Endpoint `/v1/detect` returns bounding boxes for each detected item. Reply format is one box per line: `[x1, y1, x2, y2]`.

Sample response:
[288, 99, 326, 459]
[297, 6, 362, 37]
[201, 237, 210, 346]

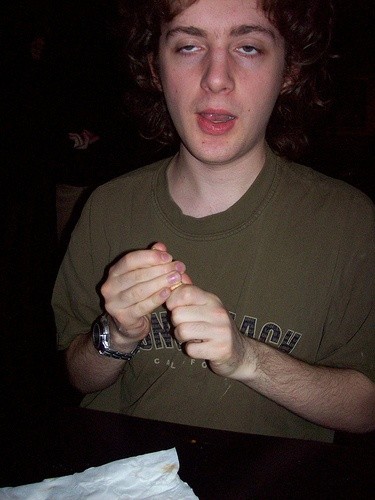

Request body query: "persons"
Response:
[51, 1, 375, 445]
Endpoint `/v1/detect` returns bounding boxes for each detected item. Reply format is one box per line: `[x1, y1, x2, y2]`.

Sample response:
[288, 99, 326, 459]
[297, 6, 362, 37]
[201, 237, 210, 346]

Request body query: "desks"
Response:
[0, 400, 375, 500]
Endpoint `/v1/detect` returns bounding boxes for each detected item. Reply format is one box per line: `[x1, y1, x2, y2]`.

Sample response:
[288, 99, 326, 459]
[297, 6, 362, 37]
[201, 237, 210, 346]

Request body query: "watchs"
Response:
[90, 313, 144, 361]
